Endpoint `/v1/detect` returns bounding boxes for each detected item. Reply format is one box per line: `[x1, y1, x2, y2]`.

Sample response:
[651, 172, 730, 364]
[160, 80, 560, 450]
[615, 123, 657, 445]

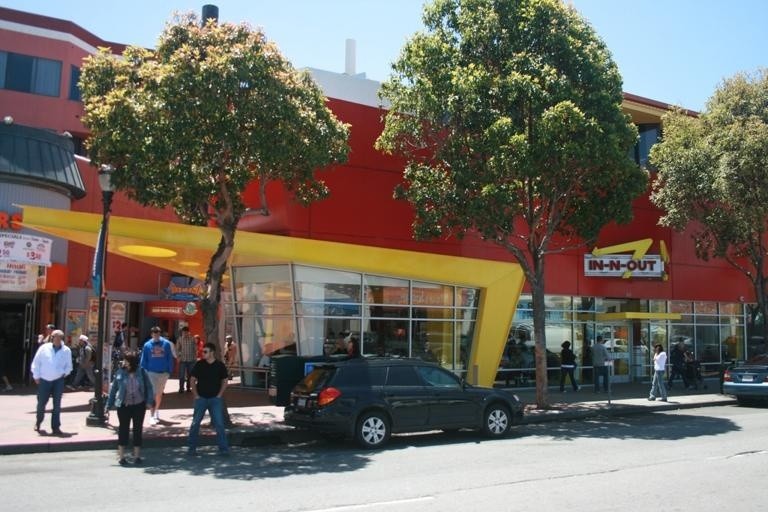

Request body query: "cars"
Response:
[281, 356, 524, 450]
[720, 352, 767, 407]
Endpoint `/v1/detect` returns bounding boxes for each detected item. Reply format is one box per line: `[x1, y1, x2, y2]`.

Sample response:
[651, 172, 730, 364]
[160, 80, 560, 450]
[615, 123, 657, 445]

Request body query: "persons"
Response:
[65, 335, 97, 392]
[224, 335, 237, 380]
[194, 334, 204, 362]
[0, 346, 13, 392]
[504, 334, 529, 385]
[667, 337, 692, 389]
[30, 329, 73, 435]
[162, 332, 170, 341]
[105, 349, 153, 465]
[344, 340, 362, 361]
[38, 324, 55, 345]
[188, 343, 232, 456]
[590, 335, 611, 394]
[560, 341, 581, 393]
[647, 344, 668, 401]
[110, 322, 128, 383]
[175, 327, 198, 394]
[140, 326, 174, 425]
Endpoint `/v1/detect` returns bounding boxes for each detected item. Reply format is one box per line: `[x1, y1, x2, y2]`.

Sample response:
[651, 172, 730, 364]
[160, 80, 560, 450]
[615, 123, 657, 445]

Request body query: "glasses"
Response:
[202, 349, 212, 354]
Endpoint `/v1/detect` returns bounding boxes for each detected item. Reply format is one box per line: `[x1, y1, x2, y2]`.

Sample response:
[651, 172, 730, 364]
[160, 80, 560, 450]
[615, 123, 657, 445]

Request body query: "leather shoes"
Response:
[53, 429, 67, 436]
[34, 424, 40, 430]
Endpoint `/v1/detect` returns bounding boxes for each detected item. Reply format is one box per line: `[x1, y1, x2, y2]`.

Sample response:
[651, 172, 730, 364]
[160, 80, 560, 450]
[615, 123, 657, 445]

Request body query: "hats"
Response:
[80, 334, 88, 342]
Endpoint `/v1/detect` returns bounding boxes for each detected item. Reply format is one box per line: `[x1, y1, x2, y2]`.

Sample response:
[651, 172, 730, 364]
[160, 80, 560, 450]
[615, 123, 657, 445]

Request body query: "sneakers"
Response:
[150, 417, 156, 426]
[218, 448, 232, 456]
[187, 447, 197, 454]
[134, 457, 143, 463]
[154, 417, 162, 424]
[118, 458, 129, 466]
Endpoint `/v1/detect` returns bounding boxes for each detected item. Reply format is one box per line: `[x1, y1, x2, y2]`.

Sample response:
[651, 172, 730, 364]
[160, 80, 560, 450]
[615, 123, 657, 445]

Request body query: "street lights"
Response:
[86, 162, 119, 425]
